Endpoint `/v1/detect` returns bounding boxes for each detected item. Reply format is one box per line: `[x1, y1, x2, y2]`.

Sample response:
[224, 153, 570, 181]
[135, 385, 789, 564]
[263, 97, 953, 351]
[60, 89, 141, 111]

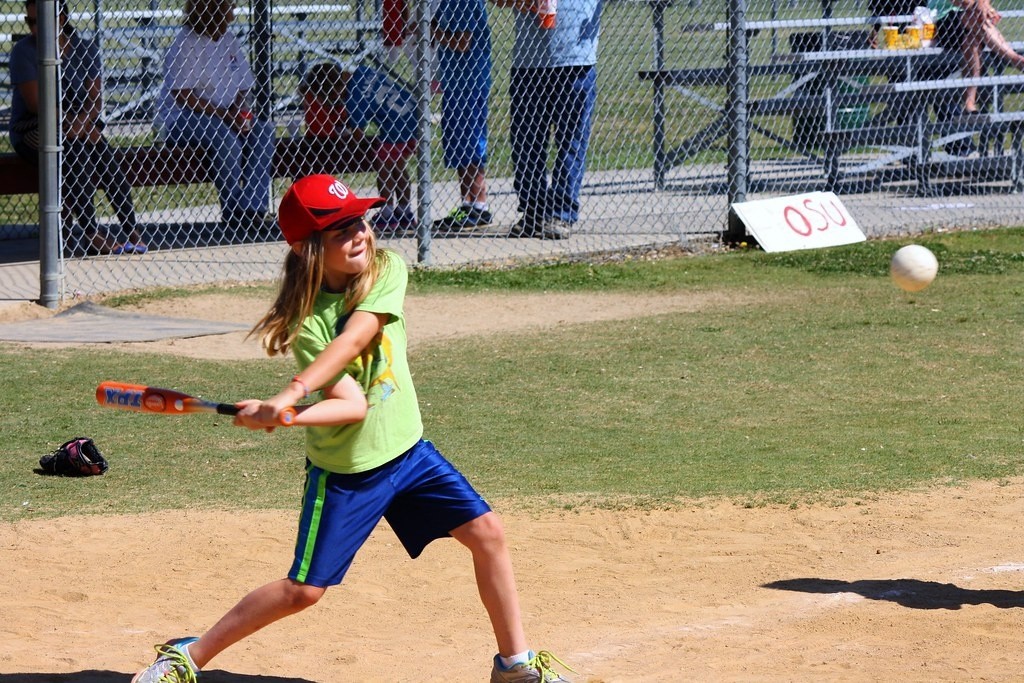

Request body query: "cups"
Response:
[882, 27, 898, 50]
[906, 25, 921, 49]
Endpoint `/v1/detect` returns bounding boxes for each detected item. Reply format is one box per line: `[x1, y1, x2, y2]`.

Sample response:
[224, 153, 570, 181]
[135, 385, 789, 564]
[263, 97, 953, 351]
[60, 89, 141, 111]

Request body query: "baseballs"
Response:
[891, 245, 939, 293]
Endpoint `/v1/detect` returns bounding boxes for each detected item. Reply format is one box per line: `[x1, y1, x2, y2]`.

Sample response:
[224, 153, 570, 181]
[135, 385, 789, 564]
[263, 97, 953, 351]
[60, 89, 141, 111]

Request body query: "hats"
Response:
[279, 173, 387, 245]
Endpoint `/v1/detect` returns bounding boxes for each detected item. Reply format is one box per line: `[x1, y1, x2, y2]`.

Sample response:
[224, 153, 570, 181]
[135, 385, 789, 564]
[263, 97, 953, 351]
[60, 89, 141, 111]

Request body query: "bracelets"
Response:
[291, 376, 310, 399]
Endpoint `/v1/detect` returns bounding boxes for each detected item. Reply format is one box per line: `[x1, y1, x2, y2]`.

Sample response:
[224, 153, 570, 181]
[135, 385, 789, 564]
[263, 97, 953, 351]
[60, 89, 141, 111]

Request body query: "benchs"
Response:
[680, 9, 1024, 191]
[0, 135, 414, 237]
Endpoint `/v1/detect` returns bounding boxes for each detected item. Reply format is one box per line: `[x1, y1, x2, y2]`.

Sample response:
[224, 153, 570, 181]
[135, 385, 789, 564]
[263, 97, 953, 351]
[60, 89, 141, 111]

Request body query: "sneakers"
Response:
[490, 650, 579, 683]
[431, 203, 493, 230]
[368, 210, 418, 234]
[131, 637, 202, 683]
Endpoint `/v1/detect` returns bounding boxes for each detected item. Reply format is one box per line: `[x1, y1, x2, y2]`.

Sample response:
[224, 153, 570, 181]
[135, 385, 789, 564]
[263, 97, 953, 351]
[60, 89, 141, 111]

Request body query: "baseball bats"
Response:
[97, 382, 297, 428]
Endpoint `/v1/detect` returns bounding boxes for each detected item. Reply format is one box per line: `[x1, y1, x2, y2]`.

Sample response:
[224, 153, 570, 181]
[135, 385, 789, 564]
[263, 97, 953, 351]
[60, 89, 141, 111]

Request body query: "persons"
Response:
[406, 0, 604, 239]
[9, 0, 148, 253]
[307, 63, 418, 229]
[154, 0, 276, 230]
[129, 173, 603, 683]
[928, 0, 1024, 113]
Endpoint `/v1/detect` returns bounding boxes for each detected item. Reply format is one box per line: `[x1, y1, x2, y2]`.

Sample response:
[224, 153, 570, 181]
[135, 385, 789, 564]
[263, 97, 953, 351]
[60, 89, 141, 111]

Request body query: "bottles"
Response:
[240, 91, 254, 132]
[537, 0, 557, 29]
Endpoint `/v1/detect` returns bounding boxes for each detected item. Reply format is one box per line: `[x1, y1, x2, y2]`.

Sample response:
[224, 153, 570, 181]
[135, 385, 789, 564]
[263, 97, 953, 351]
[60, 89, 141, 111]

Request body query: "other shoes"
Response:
[542, 218, 572, 239]
[508, 216, 544, 238]
[220, 207, 286, 244]
[85, 242, 134, 255]
[133, 245, 147, 252]
